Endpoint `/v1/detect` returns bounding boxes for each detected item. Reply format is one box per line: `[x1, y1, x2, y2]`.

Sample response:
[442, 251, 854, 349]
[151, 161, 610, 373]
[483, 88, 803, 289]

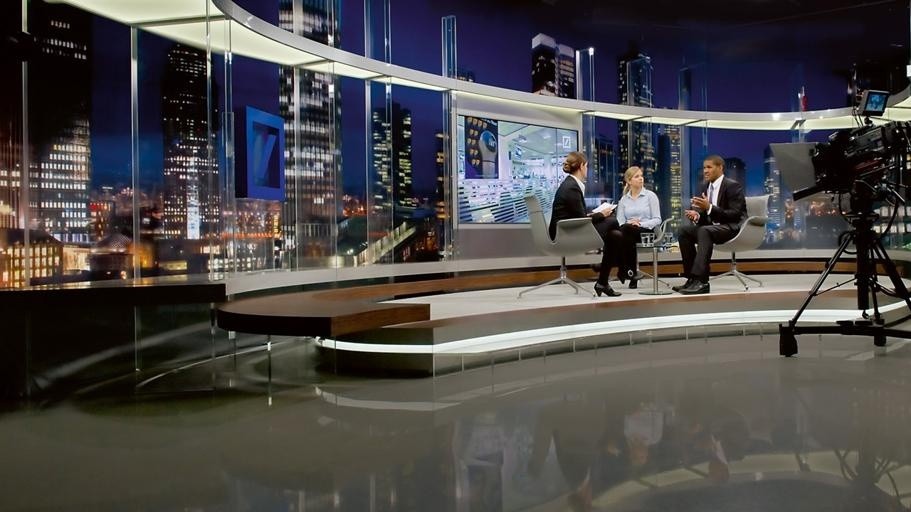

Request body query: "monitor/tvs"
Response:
[452, 113, 582, 231]
[859, 90, 889, 117]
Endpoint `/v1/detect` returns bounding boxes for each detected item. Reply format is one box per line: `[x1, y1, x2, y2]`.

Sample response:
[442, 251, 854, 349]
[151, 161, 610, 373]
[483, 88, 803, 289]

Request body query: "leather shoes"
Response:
[679, 280, 712, 295]
[618, 272, 638, 289]
[672, 277, 694, 292]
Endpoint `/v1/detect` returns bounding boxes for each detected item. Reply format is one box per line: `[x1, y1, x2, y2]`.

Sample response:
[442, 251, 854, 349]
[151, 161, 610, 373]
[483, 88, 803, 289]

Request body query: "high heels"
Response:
[594, 281, 623, 297]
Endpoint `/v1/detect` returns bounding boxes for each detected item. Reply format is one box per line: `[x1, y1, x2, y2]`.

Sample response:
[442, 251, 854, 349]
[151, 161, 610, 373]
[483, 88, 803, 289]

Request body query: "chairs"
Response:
[608, 216, 675, 288]
[518, 195, 605, 298]
[708, 193, 775, 288]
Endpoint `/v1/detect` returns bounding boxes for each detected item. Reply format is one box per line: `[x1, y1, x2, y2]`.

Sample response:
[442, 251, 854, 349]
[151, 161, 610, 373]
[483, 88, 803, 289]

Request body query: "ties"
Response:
[708, 185, 714, 223]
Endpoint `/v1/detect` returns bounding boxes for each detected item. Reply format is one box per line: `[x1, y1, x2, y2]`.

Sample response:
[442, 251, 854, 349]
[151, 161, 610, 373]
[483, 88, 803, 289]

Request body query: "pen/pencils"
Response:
[610, 201, 614, 208]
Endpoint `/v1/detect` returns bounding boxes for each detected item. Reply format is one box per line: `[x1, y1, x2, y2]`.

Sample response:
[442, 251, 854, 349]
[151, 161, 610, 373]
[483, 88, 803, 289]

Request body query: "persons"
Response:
[549, 152, 645, 297]
[615, 166, 662, 289]
[866, 95, 883, 111]
[672, 155, 748, 294]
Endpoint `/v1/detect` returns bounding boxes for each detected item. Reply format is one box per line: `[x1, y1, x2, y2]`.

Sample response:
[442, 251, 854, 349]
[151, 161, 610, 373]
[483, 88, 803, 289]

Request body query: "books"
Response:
[592, 202, 617, 214]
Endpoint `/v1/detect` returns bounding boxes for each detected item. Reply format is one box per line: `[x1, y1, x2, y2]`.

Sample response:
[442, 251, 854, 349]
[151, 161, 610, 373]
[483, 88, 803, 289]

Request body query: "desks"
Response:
[636, 246, 678, 295]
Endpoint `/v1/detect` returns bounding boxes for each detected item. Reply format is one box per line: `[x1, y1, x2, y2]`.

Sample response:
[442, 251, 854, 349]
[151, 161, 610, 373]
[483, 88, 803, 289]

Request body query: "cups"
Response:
[664, 233, 674, 245]
[641, 233, 655, 247]
